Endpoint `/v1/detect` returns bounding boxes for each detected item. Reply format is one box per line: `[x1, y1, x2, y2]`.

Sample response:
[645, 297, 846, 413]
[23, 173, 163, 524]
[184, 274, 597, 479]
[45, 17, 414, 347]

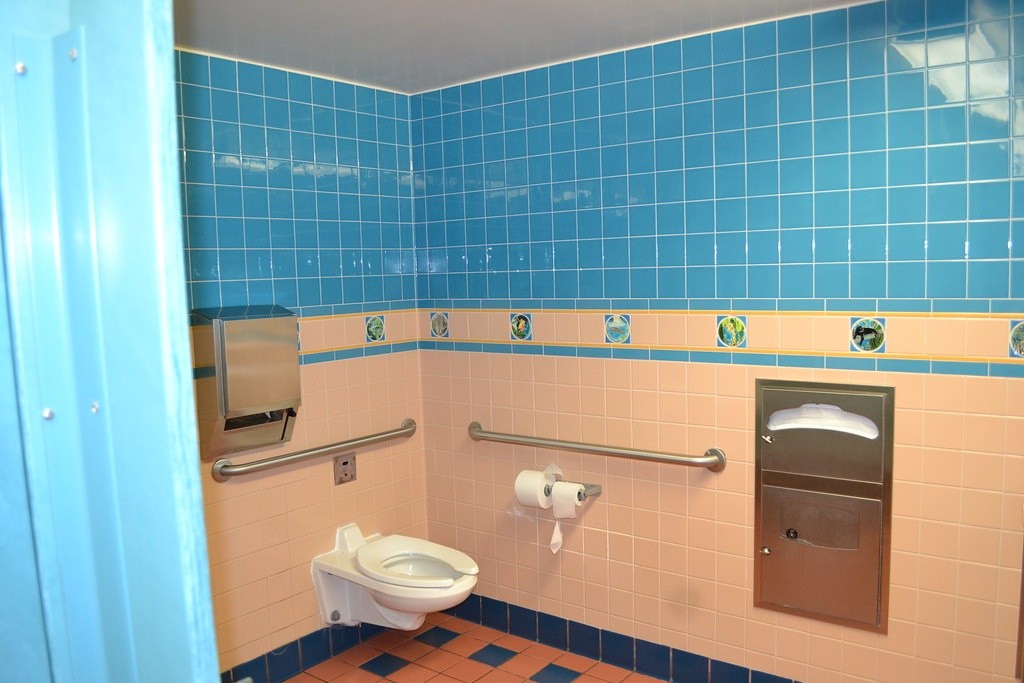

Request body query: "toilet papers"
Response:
[515, 461, 563, 509]
[550, 481, 588, 554]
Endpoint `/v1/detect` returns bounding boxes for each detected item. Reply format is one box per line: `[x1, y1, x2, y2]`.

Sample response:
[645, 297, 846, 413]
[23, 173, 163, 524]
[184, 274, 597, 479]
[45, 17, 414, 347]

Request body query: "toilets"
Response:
[311, 523, 480, 632]
